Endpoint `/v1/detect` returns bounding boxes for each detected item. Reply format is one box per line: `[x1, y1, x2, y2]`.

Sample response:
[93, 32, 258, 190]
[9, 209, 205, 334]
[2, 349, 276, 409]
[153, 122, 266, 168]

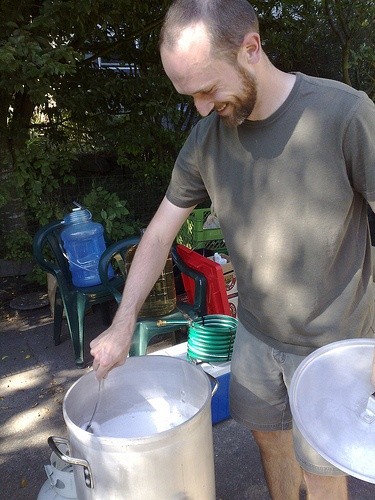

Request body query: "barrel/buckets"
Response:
[47, 355, 219, 500]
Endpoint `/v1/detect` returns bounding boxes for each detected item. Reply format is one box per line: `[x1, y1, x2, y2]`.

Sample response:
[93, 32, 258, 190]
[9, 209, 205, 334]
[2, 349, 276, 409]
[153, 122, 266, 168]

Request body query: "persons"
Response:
[89, 2, 375, 500]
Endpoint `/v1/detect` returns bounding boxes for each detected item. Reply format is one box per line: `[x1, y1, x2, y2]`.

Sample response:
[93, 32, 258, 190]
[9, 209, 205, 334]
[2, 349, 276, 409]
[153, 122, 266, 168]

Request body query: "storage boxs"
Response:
[146, 342, 231, 427]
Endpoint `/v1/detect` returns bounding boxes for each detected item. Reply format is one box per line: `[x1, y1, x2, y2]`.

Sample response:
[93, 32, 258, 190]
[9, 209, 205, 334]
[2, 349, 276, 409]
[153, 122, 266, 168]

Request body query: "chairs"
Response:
[32, 220, 236, 369]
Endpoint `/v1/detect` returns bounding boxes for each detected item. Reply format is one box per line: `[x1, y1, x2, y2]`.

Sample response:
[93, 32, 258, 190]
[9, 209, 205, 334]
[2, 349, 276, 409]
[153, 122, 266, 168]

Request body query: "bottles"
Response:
[61, 208, 114, 287]
[125, 228, 176, 317]
